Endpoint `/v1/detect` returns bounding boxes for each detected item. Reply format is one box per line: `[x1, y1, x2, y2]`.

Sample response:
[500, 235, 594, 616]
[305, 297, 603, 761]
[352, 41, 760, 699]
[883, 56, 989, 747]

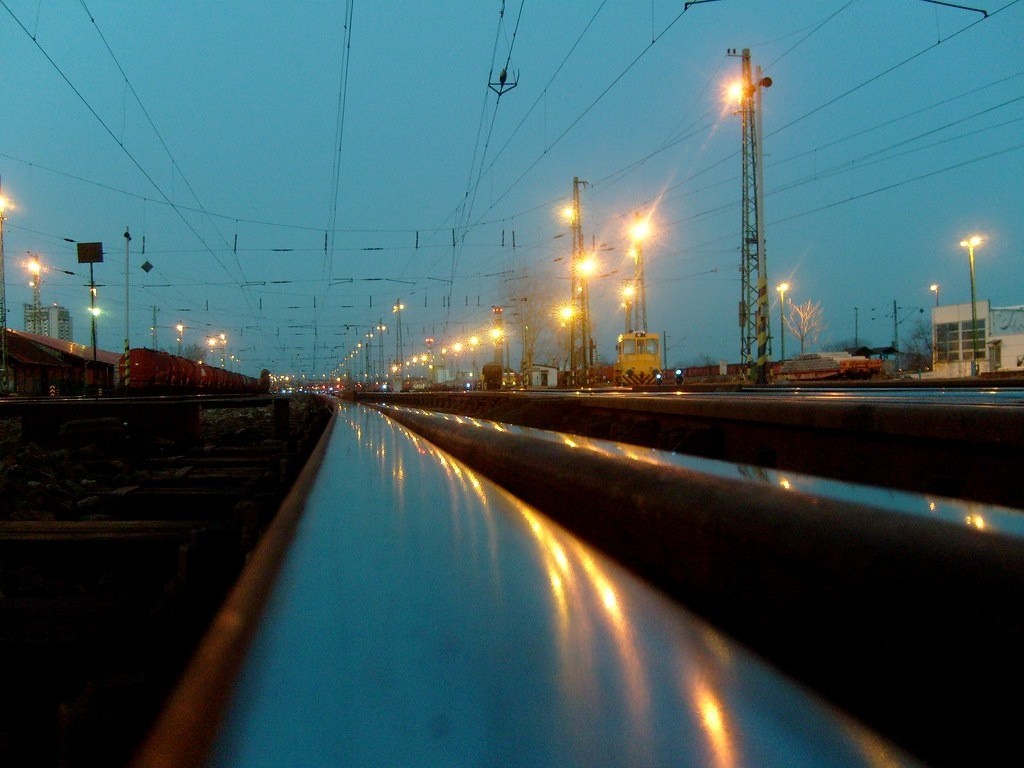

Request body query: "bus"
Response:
[613, 332, 661, 387]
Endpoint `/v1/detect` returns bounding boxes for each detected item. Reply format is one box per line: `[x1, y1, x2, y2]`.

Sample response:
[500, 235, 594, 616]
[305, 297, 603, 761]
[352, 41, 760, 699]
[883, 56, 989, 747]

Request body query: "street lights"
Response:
[0, 194, 13, 386]
[623, 215, 651, 333]
[90, 307, 101, 349]
[727, 81, 768, 388]
[777, 283, 789, 362]
[930, 284, 939, 306]
[960, 236, 983, 376]
[335, 300, 506, 393]
[560, 206, 601, 387]
[854, 306, 860, 348]
[176, 324, 184, 356]
[28, 261, 41, 335]
